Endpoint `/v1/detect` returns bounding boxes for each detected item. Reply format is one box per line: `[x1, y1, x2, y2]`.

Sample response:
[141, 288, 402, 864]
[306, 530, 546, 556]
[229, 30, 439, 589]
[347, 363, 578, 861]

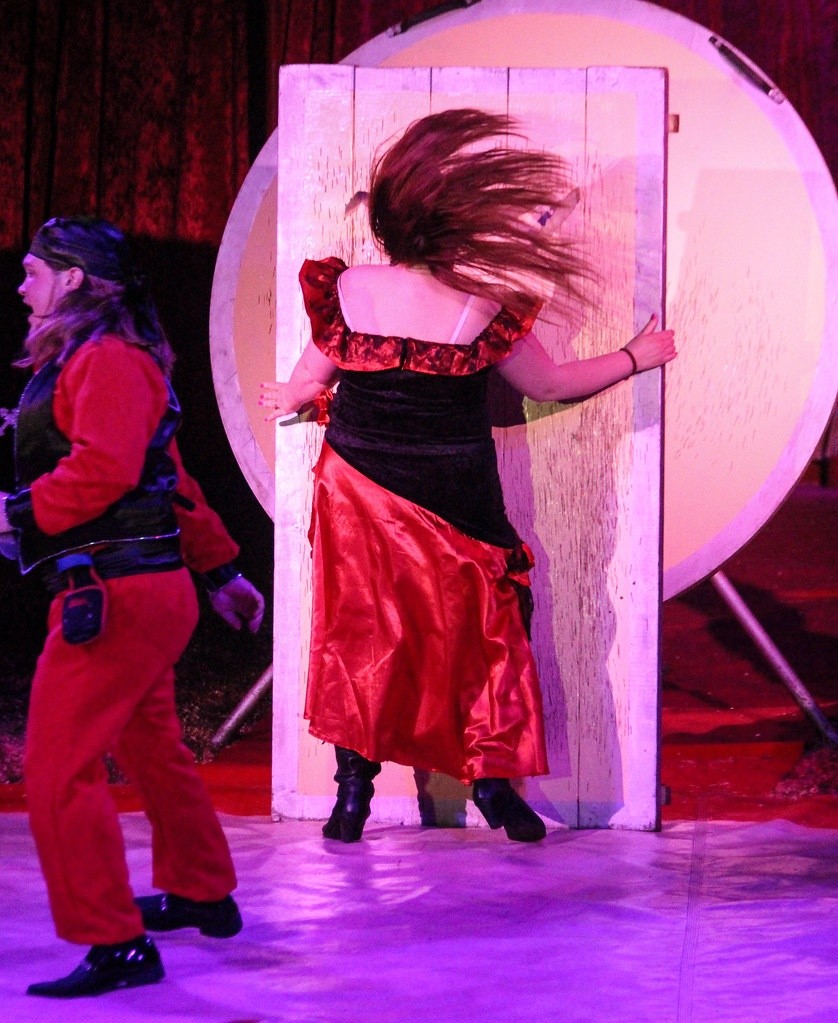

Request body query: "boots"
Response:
[322, 744, 380, 841]
[472, 777, 546, 841]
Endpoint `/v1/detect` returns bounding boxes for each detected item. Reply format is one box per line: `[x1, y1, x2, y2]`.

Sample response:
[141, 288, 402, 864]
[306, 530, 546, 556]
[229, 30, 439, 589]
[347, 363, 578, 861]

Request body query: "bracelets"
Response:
[619, 348, 637, 374]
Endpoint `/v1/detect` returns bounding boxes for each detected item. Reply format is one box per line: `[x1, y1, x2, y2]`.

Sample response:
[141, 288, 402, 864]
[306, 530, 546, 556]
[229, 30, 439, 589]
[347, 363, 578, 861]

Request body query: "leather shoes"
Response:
[26, 936, 165, 999]
[133, 892, 242, 938]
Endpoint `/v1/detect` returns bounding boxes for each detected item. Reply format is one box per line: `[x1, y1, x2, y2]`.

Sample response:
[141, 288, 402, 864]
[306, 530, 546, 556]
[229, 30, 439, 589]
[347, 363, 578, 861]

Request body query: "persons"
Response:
[256, 109, 677, 841]
[0, 212, 265, 997]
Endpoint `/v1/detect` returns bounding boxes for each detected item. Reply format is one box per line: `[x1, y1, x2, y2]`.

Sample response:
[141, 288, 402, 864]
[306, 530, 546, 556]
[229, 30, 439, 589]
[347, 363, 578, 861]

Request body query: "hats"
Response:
[31, 216, 128, 281]
